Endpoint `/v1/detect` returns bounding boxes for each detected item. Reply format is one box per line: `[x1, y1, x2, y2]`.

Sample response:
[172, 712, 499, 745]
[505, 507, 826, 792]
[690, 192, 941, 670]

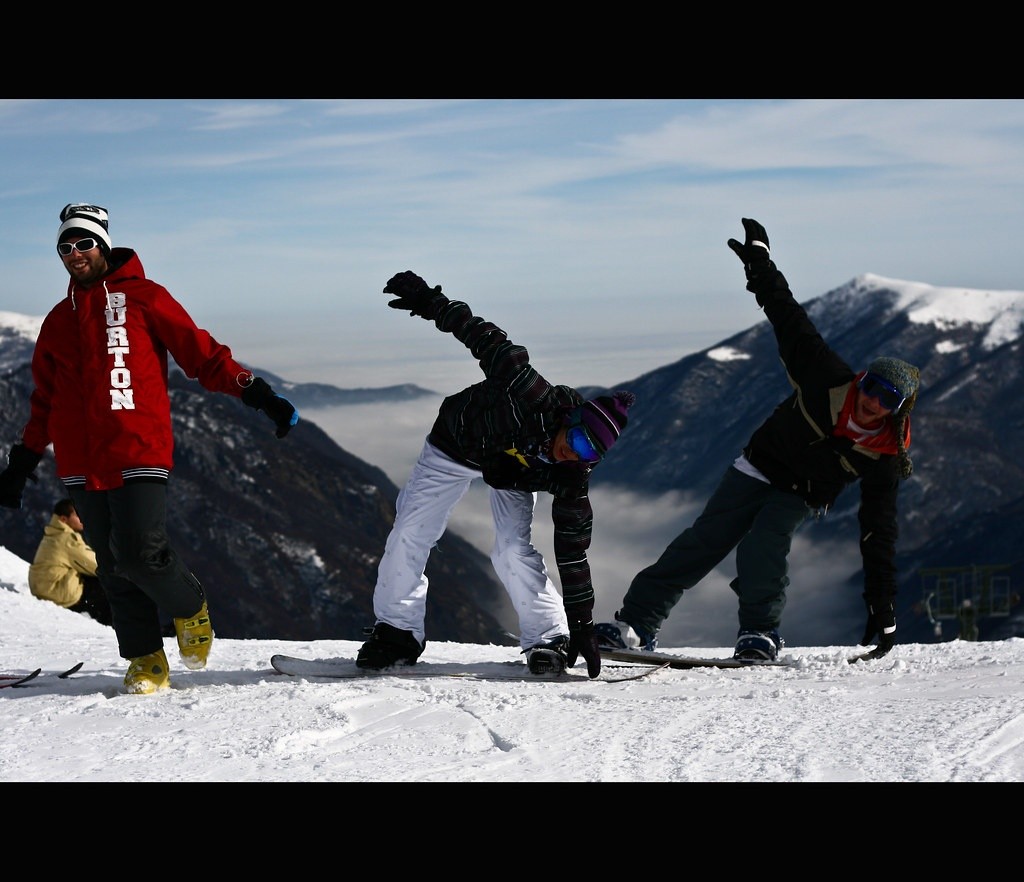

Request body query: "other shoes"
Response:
[528, 645, 565, 672]
[173, 600, 211, 660]
[735, 630, 780, 656]
[124, 655, 167, 693]
[596, 620, 655, 648]
[359, 624, 419, 666]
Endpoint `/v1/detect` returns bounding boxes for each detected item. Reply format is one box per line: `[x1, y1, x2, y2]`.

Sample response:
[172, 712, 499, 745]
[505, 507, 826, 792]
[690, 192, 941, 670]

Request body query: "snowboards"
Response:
[504, 631, 792, 671]
[270, 652, 671, 681]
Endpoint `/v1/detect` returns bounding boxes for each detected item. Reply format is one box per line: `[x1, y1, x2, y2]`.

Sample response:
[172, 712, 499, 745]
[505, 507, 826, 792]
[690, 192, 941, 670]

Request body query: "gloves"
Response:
[242, 377, 298, 438]
[566, 609, 600, 679]
[383, 270, 448, 321]
[728, 218, 769, 266]
[0, 444, 42, 509]
[862, 589, 897, 652]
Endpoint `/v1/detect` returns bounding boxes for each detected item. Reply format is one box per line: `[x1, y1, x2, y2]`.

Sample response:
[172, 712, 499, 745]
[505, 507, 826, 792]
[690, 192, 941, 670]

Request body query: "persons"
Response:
[0, 203, 300, 694]
[355, 268, 633, 677]
[614, 216, 925, 667]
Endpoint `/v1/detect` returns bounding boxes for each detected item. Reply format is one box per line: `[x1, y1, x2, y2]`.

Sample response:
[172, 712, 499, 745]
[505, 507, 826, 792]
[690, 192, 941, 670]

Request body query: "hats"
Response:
[870, 358, 919, 477]
[570, 390, 636, 448]
[56, 203, 110, 259]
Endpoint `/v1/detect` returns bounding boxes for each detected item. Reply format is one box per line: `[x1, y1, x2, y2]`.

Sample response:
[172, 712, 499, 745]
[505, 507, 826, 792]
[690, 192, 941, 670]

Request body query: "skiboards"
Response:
[3, 663, 86, 687]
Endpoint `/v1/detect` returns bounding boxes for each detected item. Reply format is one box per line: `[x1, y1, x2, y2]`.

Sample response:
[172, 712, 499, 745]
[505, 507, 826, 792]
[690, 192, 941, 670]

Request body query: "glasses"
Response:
[566, 412, 602, 466]
[58, 238, 98, 255]
[860, 372, 905, 410]
